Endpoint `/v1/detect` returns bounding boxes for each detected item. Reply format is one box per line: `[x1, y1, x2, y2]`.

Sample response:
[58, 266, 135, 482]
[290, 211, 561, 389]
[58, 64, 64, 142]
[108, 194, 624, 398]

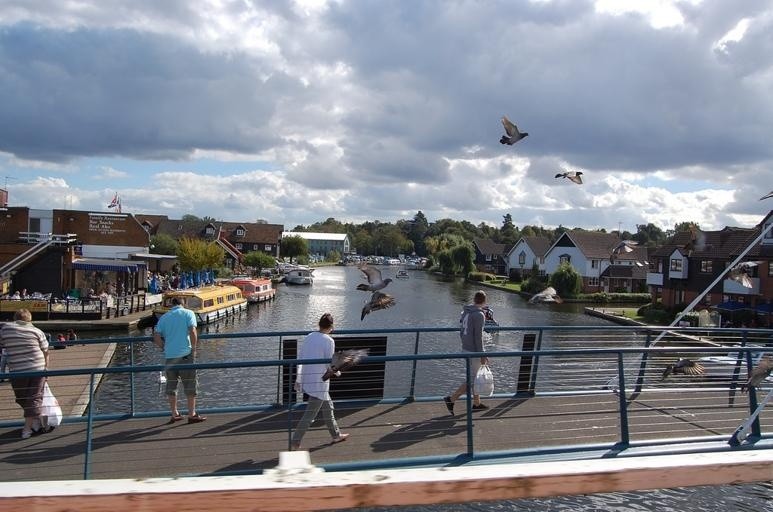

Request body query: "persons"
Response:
[0, 308, 49, 442]
[289, 314, 349, 451]
[443, 291, 489, 417]
[151, 294, 199, 425]
[43, 328, 77, 350]
[1, 266, 212, 309]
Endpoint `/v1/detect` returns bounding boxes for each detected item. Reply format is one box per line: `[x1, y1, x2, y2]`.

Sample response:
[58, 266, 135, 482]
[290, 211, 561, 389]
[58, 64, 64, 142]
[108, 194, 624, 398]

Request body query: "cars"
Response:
[243, 252, 428, 278]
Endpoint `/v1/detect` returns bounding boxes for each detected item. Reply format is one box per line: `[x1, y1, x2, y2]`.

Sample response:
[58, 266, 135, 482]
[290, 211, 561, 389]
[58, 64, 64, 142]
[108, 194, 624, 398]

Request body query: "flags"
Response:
[107, 197, 121, 212]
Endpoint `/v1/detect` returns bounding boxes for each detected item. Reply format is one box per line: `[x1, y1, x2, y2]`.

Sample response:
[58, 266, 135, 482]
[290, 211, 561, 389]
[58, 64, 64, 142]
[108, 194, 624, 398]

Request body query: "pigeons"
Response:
[723, 261, 758, 289]
[361, 292, 396, 320]
[554, 171, 584, 185]
[321, 348, 370, 381]
[661, 357, 706, 378]
[528, 286, 564, 304]
[356, 266, 394, 293]
[499, 116, 530, 145]
[741, 355, 773, 393]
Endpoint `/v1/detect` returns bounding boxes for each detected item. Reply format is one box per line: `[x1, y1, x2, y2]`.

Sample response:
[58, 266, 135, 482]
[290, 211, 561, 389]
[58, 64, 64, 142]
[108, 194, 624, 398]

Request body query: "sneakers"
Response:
[291, 445, 306, 450]
[473, 404, 488, 411]
[22, 416, 40, 439]
[333, 433, 349, 442]
[188, 413, 206, 422]
[444, 396, 454, 415]
[172, 413, 184, 420]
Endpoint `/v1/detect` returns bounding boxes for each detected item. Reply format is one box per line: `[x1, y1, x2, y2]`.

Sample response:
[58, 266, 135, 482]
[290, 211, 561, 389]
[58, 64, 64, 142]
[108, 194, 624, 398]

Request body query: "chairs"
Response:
[4, 292, 115, 313]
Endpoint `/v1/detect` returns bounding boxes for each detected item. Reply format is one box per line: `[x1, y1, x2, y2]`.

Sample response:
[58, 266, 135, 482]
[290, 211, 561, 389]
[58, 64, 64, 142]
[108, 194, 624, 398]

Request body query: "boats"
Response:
[460, 303, 499, 334]
[151, 279, 247, 326]
[224, 278, 275, 303]
[246, 294, 275, 324]
[192, 309, 247, 358]
[690, 342, 772, 386]
[283, 267, 312, 285]
[267, 274, 283, 283]
